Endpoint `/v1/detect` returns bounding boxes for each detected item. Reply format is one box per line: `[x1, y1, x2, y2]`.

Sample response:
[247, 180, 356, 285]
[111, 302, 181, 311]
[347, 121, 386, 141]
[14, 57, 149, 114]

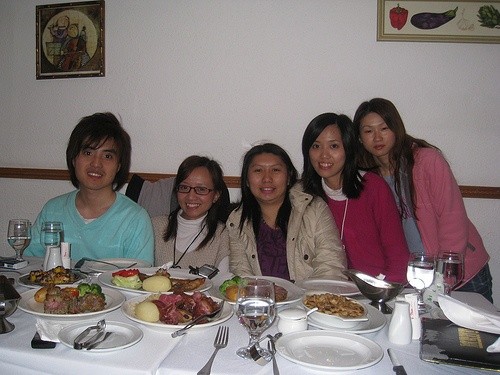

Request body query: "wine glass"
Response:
[433, 248, 465, 295]
[235, 279, 277, 362]
[406, 252, 437, 313]
[7, 219, 34, 261]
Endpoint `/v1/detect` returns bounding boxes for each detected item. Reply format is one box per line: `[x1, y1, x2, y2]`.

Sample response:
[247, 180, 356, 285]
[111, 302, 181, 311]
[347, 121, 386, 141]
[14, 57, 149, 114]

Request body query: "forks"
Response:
[82, 256, 137, 268]
[197, 325, 230, 375]
[171, 301, 224, 337]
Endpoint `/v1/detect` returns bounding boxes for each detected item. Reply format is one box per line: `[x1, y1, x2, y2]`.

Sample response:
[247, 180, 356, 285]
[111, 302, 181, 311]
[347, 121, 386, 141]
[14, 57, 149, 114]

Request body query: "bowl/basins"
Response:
[277, 308, 309, 334]
[304, 290, 368, 328]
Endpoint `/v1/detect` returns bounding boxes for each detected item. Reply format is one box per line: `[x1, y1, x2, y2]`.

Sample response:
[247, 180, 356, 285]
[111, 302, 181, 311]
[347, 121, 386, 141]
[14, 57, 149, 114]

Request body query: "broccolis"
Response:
[77, 282, 105, 300]
[219, 276, 247, 291]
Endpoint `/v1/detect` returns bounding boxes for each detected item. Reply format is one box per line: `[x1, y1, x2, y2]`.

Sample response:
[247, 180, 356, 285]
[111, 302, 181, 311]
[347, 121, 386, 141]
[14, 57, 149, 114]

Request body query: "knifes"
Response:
[387, 347, 407, 375]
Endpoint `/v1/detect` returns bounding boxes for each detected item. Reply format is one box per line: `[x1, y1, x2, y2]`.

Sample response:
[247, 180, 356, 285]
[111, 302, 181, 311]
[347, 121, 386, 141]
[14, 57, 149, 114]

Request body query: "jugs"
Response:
[341, 269, 425, 313]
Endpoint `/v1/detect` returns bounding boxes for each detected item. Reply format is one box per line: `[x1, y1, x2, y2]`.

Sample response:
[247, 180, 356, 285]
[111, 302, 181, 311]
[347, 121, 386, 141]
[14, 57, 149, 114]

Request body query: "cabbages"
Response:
[111, 274, 143, 290]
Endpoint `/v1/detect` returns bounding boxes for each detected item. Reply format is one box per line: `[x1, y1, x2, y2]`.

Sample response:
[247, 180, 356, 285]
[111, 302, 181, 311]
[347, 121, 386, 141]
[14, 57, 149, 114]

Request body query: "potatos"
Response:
[34, 287, 47, 303]
[141, 275, 171, 292]
[134, 301, 160, 322]
[225, 285, 246, 300]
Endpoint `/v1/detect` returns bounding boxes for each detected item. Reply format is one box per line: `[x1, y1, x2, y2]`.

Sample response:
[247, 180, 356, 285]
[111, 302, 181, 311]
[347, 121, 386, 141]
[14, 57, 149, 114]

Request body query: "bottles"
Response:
[44, 245, 63, 272]
[396, 294, 420, 340]
[388, 302, 413, 345]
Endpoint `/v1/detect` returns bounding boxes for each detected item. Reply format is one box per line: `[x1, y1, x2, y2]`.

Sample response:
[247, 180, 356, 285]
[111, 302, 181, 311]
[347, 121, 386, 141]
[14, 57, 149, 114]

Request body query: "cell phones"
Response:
[196, 263, 219, 280]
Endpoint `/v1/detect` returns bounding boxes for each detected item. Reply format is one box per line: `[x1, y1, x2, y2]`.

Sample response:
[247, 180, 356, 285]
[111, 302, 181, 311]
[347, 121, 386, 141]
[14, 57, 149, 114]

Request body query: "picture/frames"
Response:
[377, 0, 500, 44]
[35, 0, 105, 80]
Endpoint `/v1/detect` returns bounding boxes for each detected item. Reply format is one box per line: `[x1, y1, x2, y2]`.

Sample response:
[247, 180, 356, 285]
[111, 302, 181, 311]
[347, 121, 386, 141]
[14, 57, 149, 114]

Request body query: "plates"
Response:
[303, 302, 387, 333]
[85, 258, 152, 273]
[121, 292, 234, 329]
[97, 266, 213, 295]
[274, 329, 384, 371]
[16, 271, 91, 289]
[293, 277, 364, 297]
[221, 276, 305, 305]
[58, 320, 143, 352]
[16, 286, 126, 318]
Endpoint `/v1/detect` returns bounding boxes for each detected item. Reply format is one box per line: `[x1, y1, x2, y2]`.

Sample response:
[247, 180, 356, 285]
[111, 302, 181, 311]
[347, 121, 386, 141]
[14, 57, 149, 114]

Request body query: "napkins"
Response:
[34, 316, 77, 344]
[438, 293, 500, 352]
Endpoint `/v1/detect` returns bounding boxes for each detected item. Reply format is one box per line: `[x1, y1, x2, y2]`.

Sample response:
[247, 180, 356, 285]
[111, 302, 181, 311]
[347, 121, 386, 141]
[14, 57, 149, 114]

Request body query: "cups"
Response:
[0, 275, 22, 335]
[39, 222, 64, 250]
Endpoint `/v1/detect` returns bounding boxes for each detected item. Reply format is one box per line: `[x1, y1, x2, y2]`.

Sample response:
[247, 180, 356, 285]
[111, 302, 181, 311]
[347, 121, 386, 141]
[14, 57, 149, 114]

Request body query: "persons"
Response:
[152, 155, 229, 274]
[22, 112, 154, 267]
[226, 142, 349, 282]
[353, 98, 495, 303]
[300, 112, 410, 284]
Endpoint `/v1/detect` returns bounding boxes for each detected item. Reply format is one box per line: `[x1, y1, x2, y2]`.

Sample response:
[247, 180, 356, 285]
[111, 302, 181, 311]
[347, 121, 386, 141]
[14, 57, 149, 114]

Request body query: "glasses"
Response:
[175, 184, 215, 195]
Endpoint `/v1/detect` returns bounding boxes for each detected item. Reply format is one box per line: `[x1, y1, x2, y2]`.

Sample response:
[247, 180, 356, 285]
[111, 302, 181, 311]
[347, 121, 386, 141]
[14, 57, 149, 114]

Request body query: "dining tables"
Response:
[0, 256, 500, 375]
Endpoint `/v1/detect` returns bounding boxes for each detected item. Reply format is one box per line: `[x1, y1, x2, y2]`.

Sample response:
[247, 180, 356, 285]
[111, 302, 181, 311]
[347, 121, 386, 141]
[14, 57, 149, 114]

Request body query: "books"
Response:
[0, 256, 28, 269]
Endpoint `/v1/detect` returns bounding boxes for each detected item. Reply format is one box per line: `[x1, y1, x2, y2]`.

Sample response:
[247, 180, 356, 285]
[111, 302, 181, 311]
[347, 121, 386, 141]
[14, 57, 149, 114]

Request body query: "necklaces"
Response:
[171, 221, 207, 269]
